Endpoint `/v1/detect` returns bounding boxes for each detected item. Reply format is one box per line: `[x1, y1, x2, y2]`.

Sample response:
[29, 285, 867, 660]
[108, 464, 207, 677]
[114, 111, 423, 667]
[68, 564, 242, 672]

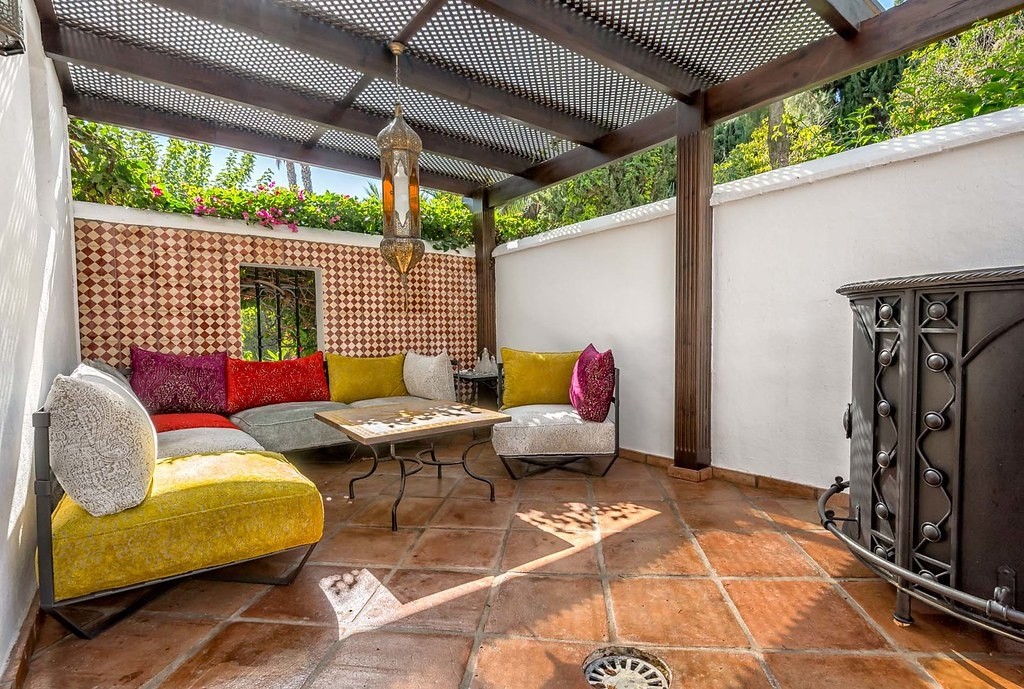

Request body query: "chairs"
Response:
[491, 362, 620, 479]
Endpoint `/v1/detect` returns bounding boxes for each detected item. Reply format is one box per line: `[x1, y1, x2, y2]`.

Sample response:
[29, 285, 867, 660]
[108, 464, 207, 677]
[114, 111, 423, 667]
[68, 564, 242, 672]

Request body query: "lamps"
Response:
[0, 0, 27, 57]
[375, 41, 426, 284]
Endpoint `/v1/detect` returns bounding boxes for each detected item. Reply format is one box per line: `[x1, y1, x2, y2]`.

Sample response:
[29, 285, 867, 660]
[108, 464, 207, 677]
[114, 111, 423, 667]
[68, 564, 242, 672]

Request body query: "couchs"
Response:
[33, 361, 436, 643]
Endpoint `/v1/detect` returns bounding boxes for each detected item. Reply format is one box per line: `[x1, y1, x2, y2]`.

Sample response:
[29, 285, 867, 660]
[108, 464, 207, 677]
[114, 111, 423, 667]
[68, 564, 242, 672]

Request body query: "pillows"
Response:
[128, 344, 228, 417]
[44, 362, 159, 517]
[225, 350, 330, 414]
[401, 346, 458, 402]
[568, 342, 615, 422]
[499, 346, 583, 410]
[324, 352, 410, 403]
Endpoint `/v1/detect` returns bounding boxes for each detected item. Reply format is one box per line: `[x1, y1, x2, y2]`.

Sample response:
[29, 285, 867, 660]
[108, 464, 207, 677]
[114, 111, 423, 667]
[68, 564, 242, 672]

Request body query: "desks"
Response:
[452, 370, 504, 438]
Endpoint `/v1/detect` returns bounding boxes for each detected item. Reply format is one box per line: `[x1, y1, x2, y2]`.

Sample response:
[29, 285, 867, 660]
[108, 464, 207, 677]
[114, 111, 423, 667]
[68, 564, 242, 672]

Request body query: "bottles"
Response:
[475, 348, 498, 375]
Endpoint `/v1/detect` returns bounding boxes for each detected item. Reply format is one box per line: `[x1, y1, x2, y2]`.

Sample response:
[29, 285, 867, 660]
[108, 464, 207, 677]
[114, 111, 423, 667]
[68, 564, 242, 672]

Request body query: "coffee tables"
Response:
[312, 399, 513, 533]
